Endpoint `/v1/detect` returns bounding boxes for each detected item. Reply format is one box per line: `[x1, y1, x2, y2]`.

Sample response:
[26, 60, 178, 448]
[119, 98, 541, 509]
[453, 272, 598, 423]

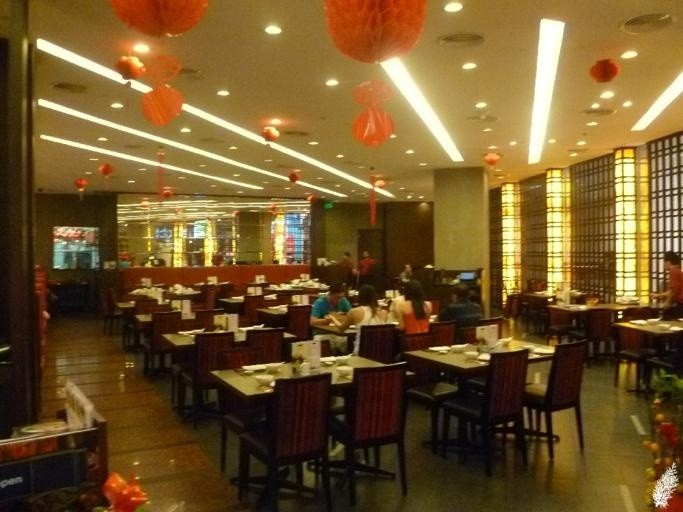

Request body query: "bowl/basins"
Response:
[254, 375, 275, 388]
[498, 338, 512, 345]
[465, 351, 479, 361]
[657, 323, 670, 332]
[451, 344, 467, 352]
[335, 366, 354, 377]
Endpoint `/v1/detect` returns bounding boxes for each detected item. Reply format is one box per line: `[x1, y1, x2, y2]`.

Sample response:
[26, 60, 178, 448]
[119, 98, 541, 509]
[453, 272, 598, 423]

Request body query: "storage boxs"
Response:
[0, 455, 79, 499]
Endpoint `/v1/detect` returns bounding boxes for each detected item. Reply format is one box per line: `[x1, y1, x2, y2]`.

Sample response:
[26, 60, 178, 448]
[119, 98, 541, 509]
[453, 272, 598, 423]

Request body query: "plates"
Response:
[628, 319, 648, 326]
[531, 348, 555, 355]
[19, 421, 70, 435]
[241, 365, 268, 371]
[428, 346, 450, 351]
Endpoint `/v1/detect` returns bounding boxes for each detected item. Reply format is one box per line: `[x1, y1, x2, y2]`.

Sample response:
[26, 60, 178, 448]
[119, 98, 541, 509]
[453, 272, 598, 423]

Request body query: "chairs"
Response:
[534, 295, 556, 337]
[143, 311, 181, 376]
[180, 332, 235, 425]
[577, 293, 605, 303]
[124, 298, 158, 350]
[569, 309, 611, 369]
[547, 304, 575, 345]
[397, 332, 457, 453]
[480, 315, 505, 338]
[614, 315, 660, 398]
[283, 340, 332, 361]
[240, 373, 333, 512]
[246, 328, 284, 363]
[359, 324, 396, 363]
[443, 348, 528, 479]
[219, 345, 265, 472]
[201, 284, 216, 300]
[525, 340, 589, 459]
[465, 324, 502, 343]
[333, 361, 408, 507]
[287, 304, 312, 339]
[625, 307, 653, 320]
[195, 309, 225, 331]
[429, 321, 457, 346]
[100, 288, 123, 336]
[646, 330, 682, 399]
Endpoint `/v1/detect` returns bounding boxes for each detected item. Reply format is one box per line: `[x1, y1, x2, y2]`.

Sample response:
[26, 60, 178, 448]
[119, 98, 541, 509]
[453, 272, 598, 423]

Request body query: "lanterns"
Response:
[289, 173, 300, 182]
[354, 108, 395, 147]
[588, 59, 619, 83]
[233, 211, 240, 216]
[485, 153, 500, 166]
[306, 194, 318, 203]
[326, 0, 424, 65]
[116, 56, 145, 79]
[111, 0, 209, 38]
[262, 126, 280, 140]
[163, 190, 173, 198]
[74, 178, 87, 187]
[142, 87, 184, 125]
[99, 164, 112, 175]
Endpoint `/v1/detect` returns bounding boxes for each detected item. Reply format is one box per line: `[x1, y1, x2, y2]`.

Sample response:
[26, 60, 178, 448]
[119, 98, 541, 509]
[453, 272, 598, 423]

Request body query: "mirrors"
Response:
[53, 226, 100, 269]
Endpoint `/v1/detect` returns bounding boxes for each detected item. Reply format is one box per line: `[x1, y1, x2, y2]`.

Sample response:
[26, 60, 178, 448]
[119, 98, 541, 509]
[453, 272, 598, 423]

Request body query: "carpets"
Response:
[100, 312, 683, 512]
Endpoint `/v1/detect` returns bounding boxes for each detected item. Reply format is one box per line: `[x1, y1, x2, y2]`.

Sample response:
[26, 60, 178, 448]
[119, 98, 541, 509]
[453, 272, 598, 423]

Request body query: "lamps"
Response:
[627, 55, 683, 131]
[36, 39, 394, 201]
[529, 19, 564, 164]
[41, 130, 263, 229]
[380, 54, 464, 162]
[37, 98, 348, 211]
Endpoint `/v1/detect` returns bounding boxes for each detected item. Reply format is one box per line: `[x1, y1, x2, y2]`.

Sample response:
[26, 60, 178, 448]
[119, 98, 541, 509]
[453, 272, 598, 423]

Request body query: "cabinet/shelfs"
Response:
[120, 266, 312, 296]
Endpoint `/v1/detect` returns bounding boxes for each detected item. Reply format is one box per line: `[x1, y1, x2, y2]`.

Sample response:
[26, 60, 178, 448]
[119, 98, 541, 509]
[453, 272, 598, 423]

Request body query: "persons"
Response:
[327, 285, 390, 355]
[131, 257, 141, 267]
[393, 281, 433, 333]
[211, 252, 225, 267]
[387, 280, 411, 320]
[157, 259, 165, 266]
[435, 282, 483, 341]
[358, 250, 375, 290]
[336, 251, 352, 283]
[308, 279, 352, 339]
[647, 249, 683, 314]
[399, 262, 411, 286]
[118, 259, 129, 269]
